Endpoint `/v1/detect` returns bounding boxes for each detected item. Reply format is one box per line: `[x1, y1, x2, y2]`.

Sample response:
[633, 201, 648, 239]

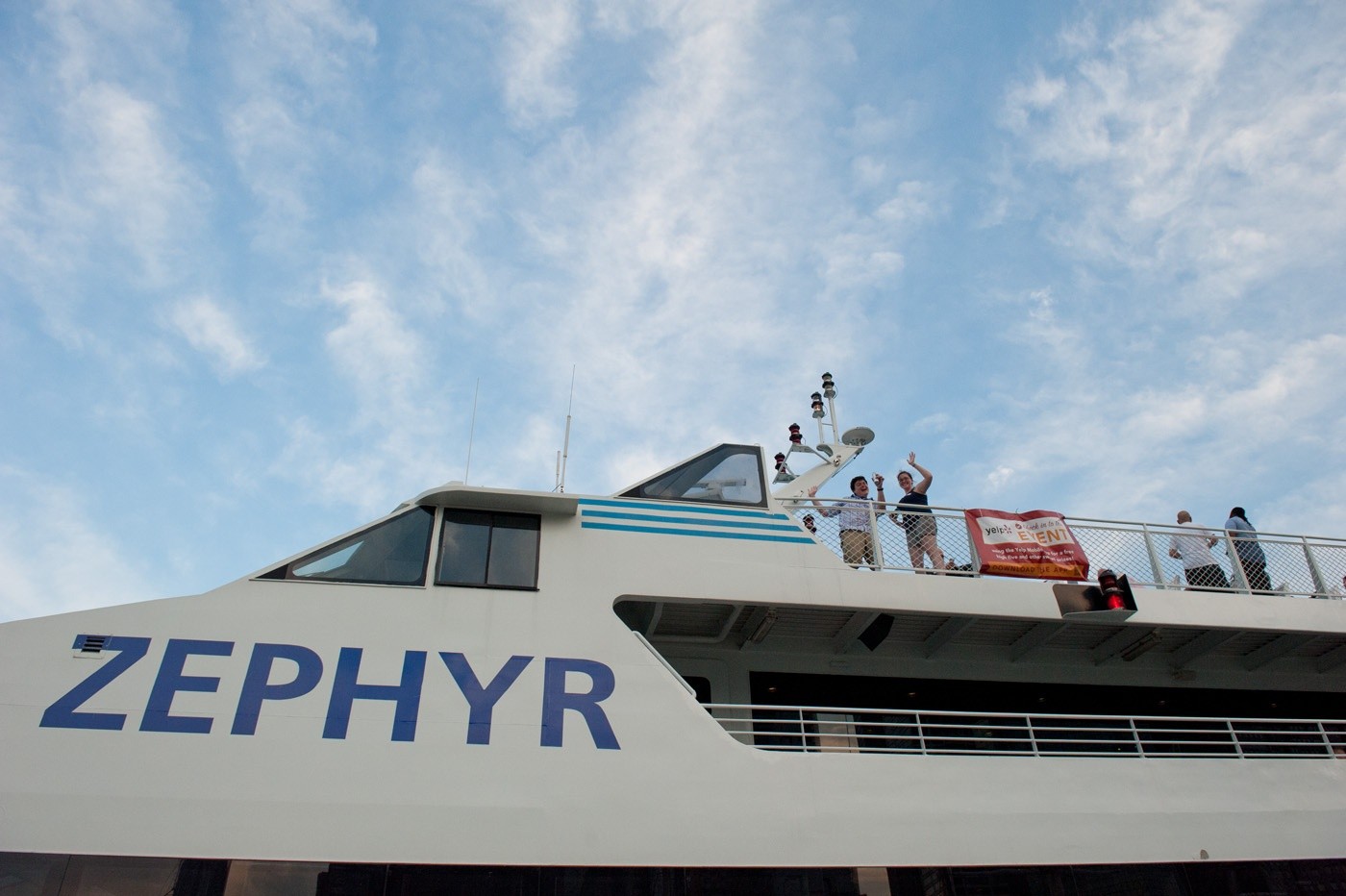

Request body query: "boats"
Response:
[2, 370, 1345, 896]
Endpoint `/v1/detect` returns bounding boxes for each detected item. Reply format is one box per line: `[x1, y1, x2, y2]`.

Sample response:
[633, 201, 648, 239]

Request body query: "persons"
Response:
[1168, 511, 1236, 594]
[1224, 507, 1275, 595]
[888, 451, 946, 576]
[807, 471, 886, 571]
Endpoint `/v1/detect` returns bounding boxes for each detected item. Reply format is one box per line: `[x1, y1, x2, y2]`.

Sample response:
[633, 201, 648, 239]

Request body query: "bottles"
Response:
[1099, 570, 1126, 610]
[872, 472, 879, 484]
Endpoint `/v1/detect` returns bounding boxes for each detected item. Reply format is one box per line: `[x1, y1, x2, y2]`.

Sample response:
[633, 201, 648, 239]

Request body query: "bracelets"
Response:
[877, 488, 883, 491]
[912, 463, 916, 467]
